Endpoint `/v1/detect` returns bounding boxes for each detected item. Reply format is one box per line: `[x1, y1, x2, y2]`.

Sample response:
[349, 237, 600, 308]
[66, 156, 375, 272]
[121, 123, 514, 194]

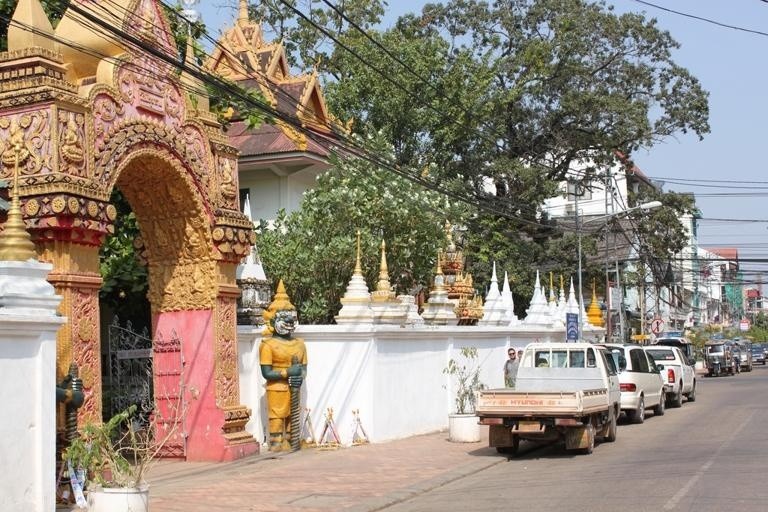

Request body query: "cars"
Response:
[593, 337, 697, 423]
[705, 338, 768, 376]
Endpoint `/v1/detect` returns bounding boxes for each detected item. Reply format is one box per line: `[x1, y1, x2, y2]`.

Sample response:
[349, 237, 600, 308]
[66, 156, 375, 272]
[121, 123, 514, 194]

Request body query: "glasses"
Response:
[509, 353, 515, 356]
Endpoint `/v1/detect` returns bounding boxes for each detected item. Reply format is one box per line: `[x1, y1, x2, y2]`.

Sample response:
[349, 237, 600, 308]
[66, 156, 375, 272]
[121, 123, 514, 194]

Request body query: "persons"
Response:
[730, 343, 743, 373]
[503, 346, 519, 388]
[517, 348, 524, 364]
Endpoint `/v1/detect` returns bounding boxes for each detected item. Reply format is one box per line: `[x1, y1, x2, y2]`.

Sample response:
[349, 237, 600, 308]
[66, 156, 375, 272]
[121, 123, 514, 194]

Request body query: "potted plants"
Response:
[441, 347, 490, 443]
[64, 386, 202, 512]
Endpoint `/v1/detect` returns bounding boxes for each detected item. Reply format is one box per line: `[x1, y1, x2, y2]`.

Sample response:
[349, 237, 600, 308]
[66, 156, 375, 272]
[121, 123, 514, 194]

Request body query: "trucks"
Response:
[476, 342, 622, 454]
